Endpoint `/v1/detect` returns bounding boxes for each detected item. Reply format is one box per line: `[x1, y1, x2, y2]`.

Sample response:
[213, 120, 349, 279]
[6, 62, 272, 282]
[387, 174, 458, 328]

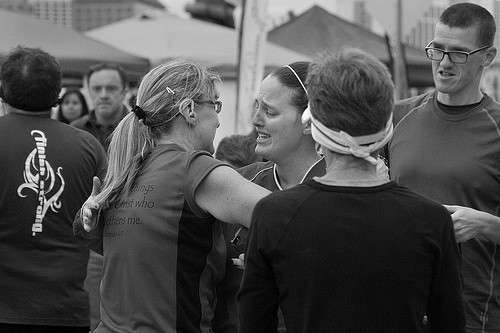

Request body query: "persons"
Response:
[0, 46, 132, 333]
[383, 3, 500, 332]
[72, 47, 471, 333]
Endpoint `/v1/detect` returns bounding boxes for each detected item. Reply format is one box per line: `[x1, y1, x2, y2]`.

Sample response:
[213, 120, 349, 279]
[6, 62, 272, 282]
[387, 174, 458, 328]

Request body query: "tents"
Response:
[266, 4, 437, 87]
[85, 9, 315, 81]
[1, 4, 150, 88]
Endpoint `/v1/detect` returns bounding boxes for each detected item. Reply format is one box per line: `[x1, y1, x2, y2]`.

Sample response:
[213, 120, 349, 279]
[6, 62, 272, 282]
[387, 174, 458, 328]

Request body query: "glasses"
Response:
[424, 39, 491, 64]
[194, 100, 223, 115]
